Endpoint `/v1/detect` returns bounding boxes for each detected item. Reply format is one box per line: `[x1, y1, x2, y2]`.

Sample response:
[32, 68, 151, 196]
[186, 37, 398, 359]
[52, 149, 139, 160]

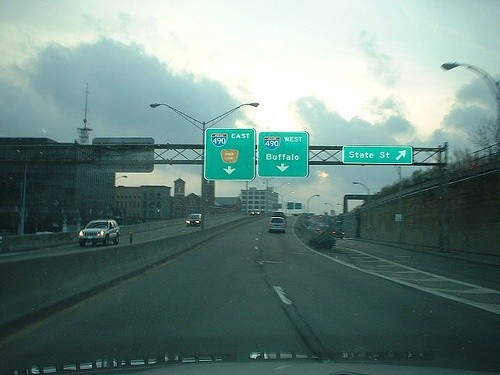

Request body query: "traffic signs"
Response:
[202, 127, 257, 181]
[340, 145, 413, 166]
[256, 131, 310, 179]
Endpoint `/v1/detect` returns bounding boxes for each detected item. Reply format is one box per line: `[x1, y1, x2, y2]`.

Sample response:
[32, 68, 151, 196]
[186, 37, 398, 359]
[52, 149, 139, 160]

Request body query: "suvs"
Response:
[330, 229, 344, 239]
[78, 219, 120, 248]
[268, 217, 286, 233]
[186, 213, 203, 226]
[272, 212, 286, 221]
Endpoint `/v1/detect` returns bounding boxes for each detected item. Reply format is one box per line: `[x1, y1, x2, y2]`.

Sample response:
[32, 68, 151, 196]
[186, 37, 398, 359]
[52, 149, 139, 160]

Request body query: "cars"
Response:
[250, 209, 260, 216]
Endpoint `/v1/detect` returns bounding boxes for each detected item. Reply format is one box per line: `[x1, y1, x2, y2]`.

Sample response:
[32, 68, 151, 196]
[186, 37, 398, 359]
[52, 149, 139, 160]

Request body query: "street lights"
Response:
[148, 101, 262, 207]
[325, 202, 340, 215]
[275, 181, 291, 192]
[307, 194, 320, 213]
[352, 181, 371, 240]
